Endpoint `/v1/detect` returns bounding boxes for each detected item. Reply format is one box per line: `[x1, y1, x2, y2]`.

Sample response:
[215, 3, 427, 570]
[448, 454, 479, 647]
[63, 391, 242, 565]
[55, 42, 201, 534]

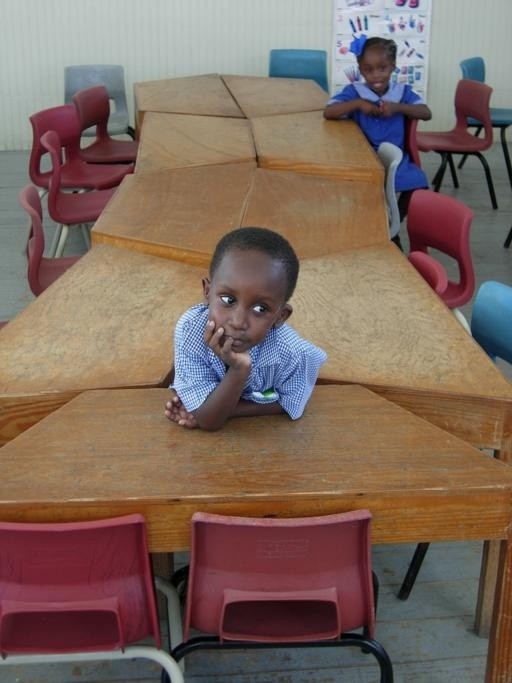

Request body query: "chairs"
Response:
[396, 280, 512, 602]
[458, 57, 511, 180]
[73, 86, 137, 164]
[408, 188, 475, 336]
[41, 130, 117, 258]
[2, 513, 186, 681]
[376, 141, 404, 251]
[28, 103, 133, 198]
[63, 64, 129, 136]
[269, 48, 329, 93]
[164, 502, 394, 682]
[417, 80, 499, 211]
[405, 115, 422, 170]
[407, 251, 447, 293]
[19, 185, 81, 296]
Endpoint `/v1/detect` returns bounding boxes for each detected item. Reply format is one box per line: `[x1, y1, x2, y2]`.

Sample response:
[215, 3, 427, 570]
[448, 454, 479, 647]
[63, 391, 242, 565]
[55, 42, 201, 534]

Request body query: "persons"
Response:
[163, 227, 329, 431]
[323, 37, 432, 255]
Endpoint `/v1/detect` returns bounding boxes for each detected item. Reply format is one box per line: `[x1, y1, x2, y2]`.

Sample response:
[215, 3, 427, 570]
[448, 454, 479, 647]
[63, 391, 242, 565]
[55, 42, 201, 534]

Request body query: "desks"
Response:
[0, 384, 511, 683]
[220, 73, 330, 121]
[91, 161, 256, 268]
[133, 73, 246, 143]
[286, 242, 511, 640]
[1, 244, 210, 386]
[134, 110, 257, 173]
[241, 166, 390, 259]
[250, 110, 386, 181]
